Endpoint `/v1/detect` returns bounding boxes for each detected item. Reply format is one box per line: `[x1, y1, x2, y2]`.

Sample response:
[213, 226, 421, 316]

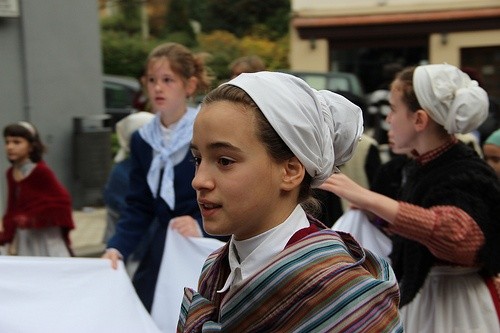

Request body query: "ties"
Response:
[140, 108, 199, 209]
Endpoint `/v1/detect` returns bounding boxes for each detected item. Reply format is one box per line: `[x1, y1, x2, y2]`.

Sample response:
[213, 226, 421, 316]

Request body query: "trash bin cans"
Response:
[72, 112, 116, 208]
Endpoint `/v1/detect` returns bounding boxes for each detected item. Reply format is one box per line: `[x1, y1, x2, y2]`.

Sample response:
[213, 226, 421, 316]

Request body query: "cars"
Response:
[273, 68, 366, 103]
[102, 72, 147, 129]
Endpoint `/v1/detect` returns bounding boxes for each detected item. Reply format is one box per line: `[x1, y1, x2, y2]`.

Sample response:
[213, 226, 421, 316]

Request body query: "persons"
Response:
[304, 88, 500, 320]
[309, 63, 500, 333]
[172, 72, 404, 332]
[0, 122, 78, 257]
[97, 41, 267, 314]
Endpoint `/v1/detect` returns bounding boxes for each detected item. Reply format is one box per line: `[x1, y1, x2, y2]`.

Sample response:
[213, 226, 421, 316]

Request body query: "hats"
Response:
[219, 70, 364, 189]
[415, 63, 488, 158]
